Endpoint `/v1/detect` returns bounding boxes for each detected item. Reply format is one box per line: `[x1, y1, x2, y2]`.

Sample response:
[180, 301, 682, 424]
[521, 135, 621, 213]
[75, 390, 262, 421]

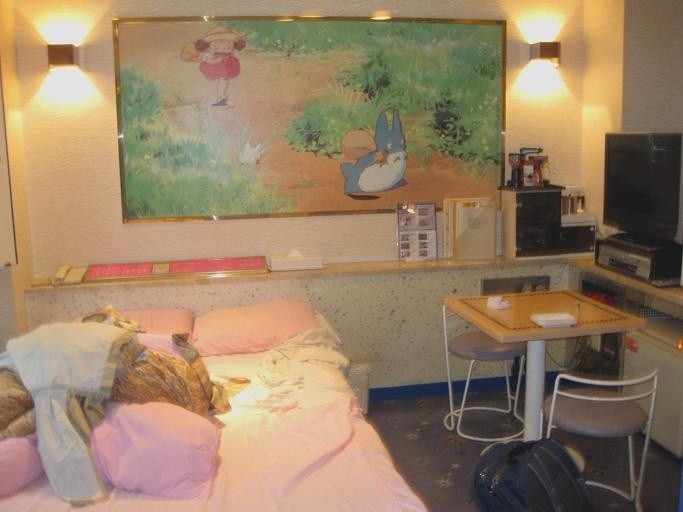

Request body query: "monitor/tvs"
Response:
[602, 129, 682, 250]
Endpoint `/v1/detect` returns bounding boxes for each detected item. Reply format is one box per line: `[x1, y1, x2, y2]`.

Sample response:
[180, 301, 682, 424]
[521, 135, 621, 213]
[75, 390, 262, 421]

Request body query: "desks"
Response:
[570, 259, 683, 461]
[445, 290, 646, 473]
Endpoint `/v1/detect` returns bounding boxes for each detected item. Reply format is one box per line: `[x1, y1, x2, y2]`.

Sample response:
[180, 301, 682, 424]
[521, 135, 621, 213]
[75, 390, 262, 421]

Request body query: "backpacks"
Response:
[468, 435, 599, 512]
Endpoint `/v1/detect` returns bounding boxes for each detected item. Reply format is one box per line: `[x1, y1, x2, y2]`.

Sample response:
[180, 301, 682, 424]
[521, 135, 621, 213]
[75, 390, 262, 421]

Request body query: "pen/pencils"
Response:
[575, 302, 580, 322]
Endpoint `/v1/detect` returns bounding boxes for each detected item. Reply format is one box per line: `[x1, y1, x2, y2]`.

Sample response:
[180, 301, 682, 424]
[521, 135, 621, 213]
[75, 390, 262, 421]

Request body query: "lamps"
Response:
[530, 42, 560, 69]
[47, 43, 77, 67]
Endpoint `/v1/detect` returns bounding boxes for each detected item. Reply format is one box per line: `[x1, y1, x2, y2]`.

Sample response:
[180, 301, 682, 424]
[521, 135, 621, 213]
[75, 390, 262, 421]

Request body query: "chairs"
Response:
[442, 305, 524, 442]
[539, 369, 659, 512]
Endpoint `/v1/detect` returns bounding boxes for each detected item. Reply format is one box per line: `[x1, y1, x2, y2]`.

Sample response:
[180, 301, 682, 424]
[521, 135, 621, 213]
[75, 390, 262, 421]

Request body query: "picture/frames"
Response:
[111, 16, 507, 225]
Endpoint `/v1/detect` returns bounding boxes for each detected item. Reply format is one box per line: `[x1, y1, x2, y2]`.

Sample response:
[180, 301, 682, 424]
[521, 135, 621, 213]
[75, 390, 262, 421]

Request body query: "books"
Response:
[443, 198, 497, 260]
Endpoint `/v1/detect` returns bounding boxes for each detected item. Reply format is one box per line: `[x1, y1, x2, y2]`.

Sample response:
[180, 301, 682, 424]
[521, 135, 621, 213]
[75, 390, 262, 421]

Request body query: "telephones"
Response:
[54, 264, 88, 287]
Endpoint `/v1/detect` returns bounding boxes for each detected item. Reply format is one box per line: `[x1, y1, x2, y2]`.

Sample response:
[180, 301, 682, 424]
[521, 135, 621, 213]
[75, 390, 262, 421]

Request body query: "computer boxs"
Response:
[594, 237, 683, 288]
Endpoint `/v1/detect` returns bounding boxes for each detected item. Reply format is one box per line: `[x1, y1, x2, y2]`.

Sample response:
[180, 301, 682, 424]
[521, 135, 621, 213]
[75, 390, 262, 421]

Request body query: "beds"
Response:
[0, 300, 427, 512]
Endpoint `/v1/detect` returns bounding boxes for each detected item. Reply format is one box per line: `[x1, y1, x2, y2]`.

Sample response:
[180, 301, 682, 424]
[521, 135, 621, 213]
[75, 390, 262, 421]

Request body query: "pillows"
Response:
[117, 308, 193, 341]
[189, 300, 317, 356]
